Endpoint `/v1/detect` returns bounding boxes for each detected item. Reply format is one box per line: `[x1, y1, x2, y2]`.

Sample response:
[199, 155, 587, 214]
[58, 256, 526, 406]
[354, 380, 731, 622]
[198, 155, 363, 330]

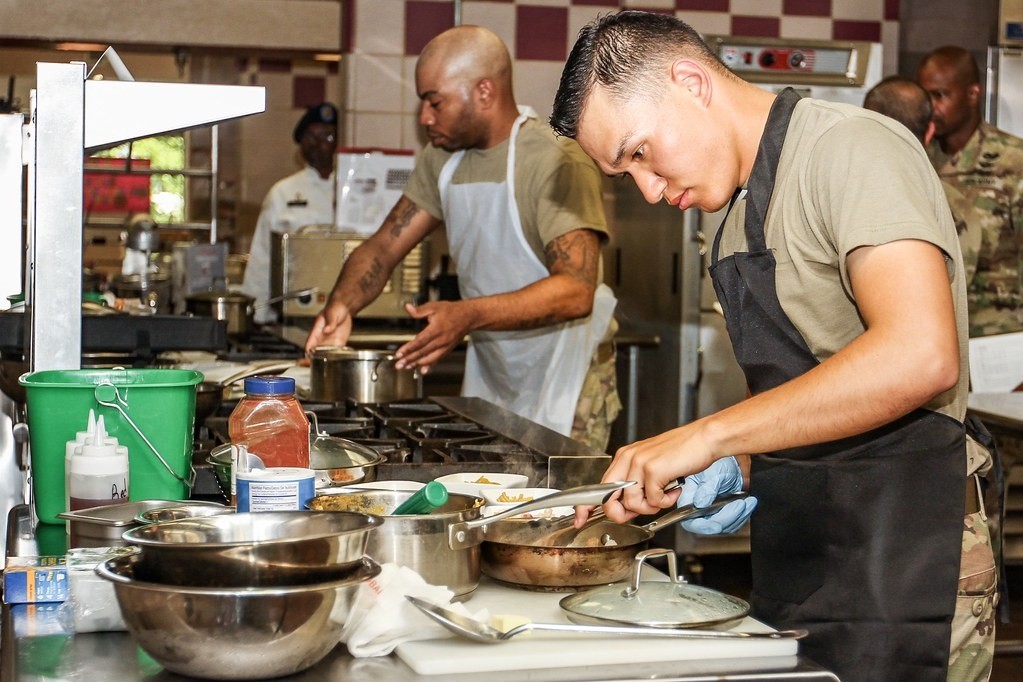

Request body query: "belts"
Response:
[589, 342, 614, 367]
[964, 474, 987, 516]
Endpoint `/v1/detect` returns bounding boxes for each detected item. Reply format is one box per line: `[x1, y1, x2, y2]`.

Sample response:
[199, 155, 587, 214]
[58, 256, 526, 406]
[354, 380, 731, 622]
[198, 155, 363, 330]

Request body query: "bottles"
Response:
[66, 409, 129, 548]
[227, 375, 316, 514]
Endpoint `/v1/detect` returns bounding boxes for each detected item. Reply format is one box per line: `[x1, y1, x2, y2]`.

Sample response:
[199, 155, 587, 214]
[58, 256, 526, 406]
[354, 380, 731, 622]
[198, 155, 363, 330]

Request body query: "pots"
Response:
[304, 480, 638, 596]
[204, 411, 388, 505]
[195, 361, 298, 429]
[183, 275, 320, 336]
[309, 344, 424, 404]
[480, 491, 750, 586]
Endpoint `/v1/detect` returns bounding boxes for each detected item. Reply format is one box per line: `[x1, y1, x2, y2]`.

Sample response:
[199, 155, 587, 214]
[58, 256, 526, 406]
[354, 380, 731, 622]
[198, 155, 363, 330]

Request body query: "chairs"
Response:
[967, 330, 1023, 469]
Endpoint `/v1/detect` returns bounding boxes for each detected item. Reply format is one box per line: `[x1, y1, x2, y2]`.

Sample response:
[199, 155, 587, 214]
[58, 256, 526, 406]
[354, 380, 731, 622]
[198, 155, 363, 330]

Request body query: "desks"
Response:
[968, 391, 1023, 429]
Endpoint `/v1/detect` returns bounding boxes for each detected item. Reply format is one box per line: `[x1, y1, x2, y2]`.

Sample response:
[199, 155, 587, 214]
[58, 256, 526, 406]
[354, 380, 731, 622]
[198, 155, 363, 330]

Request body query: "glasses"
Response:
[306, 134, 335, 144]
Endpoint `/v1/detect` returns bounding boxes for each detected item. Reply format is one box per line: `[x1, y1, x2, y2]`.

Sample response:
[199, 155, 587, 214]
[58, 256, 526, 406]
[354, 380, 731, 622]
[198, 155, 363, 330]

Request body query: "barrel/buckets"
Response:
[18, 364, 206, 524]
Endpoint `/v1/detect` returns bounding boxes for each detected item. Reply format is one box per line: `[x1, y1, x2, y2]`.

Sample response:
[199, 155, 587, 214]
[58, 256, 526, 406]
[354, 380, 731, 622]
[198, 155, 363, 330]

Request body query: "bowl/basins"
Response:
[121, 509, 385, 588]
[481, 488, 562, 506]
[55, 498, 234, 550]
[94, 550, 382, 682]
[483, 504, 576, 520]
[434, 471, 529, 488]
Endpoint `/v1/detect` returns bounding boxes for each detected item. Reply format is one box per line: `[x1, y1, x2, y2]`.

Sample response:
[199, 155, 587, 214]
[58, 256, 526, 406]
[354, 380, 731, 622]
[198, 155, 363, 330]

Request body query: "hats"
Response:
[294, 103, 338, 142]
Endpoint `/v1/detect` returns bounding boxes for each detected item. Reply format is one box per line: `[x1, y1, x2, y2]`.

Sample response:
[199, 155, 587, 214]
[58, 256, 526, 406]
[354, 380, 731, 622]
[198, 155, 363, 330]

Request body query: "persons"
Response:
[863, 76, 981, 291]
[304, 26, 623, 452]
[243, 101, 336, 324]
[547, 10, 1000, 681]
[914, 46, 1022, 339]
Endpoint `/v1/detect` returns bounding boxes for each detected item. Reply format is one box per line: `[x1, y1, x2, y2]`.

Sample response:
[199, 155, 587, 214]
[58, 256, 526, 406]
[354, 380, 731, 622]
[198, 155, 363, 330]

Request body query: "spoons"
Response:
[404, 593, 809, 644]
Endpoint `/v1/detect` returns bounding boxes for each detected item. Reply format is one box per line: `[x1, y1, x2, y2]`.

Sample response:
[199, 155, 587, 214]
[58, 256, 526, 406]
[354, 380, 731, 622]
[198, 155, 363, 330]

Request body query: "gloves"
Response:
[677, 456, 758, 535]
